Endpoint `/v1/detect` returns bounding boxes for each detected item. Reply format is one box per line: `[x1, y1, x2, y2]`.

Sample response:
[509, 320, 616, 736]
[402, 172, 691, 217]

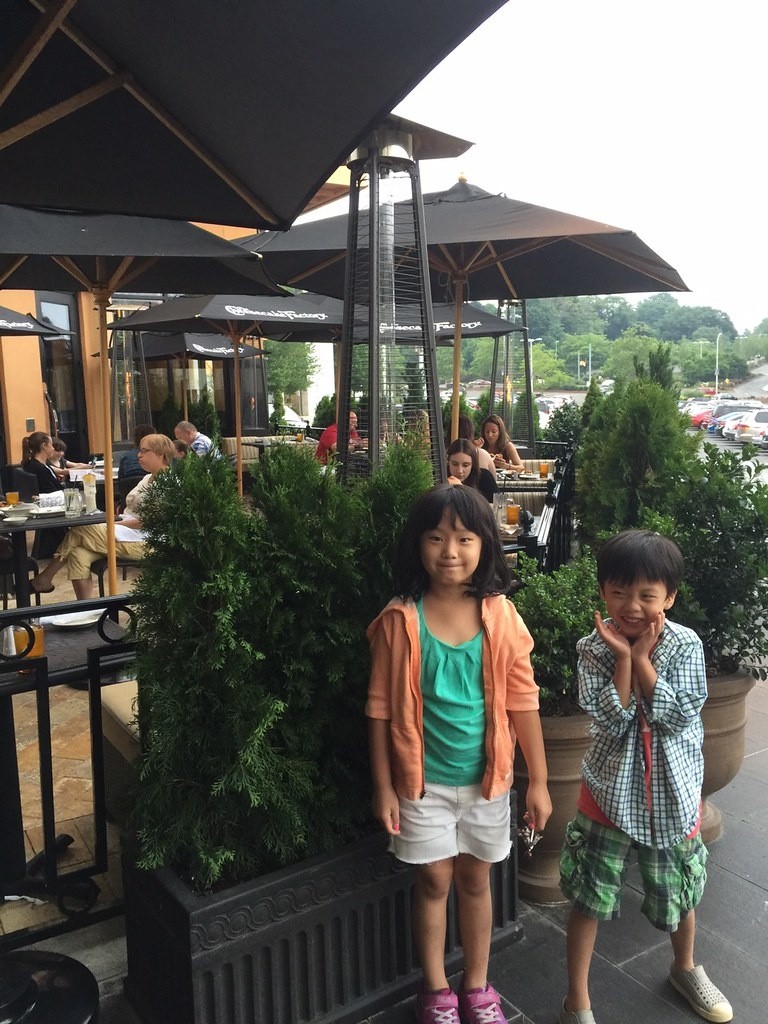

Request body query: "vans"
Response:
[734, 409, 768, 447]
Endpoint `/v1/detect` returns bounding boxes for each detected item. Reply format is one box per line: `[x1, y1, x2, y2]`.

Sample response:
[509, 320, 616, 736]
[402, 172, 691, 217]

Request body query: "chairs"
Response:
[0, 463, 145, 609]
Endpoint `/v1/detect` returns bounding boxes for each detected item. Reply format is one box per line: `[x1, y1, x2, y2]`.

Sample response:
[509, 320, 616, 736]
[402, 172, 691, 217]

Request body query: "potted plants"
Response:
[507, 437, 768, 900]
[122, 432, 526, 1024]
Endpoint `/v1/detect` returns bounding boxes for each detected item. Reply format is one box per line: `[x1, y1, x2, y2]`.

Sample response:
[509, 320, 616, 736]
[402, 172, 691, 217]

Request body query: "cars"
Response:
[597, 379, 614, 396]
[440, 379, 468, 404]
[267, 404, 308, 428]
[676, 393, 768, 450]
[468, 379, 492, 387]
[466, 391, 576, 418]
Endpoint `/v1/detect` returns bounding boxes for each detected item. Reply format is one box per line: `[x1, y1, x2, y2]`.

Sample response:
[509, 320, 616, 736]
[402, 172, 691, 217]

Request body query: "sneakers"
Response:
[413, 981, 508, 1024]
[668, 960, 733, 1023]
[560, 995, 597, 1024]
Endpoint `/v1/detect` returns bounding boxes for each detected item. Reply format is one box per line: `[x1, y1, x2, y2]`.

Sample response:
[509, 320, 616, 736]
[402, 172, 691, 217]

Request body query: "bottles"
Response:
[3, 626, 16, 655]
[497, 505, 504, 526]
[84, 474, 96, 511]
[305, 421, 312, 437]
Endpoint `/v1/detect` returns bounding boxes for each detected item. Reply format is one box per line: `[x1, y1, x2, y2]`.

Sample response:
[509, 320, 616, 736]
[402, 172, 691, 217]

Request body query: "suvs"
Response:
[713, 403, 766, 417]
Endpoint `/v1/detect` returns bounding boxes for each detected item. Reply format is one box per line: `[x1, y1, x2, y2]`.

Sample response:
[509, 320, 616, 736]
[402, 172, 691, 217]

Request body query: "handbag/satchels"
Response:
[38, 489, 65, 507]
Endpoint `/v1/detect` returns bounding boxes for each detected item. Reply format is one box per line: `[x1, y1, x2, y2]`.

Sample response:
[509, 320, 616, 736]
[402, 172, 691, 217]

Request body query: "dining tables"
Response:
[496, 472, 554, 491]
[499, 515, 540, 545]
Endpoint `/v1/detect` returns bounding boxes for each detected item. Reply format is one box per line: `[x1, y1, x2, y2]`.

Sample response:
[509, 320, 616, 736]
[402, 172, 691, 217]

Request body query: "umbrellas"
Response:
[90, 293, 528, 503]
[1, 0, 509, 231]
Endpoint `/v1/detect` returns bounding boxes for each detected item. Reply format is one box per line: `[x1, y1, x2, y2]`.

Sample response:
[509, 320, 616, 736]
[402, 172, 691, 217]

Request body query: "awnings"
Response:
[225, 180, 692, 441]
[0, 305, 77, 335]
[0, 206, 297, 597]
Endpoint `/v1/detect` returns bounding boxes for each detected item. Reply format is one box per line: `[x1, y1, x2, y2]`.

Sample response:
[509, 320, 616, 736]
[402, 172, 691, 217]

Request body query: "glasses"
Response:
[138, 448, 154, 454]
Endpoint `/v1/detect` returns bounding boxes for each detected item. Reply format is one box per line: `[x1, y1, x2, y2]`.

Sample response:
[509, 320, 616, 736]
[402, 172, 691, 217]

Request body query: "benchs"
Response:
[222, 434, 320, 473]
[496, 458, 557, 563]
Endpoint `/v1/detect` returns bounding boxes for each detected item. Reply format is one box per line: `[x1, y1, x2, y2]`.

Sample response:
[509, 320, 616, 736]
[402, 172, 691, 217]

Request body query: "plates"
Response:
[1, 509, 33, 516]
[29, 507, 65, 517]
[3, 518, 27, 525]
[53, 616, 108, 628]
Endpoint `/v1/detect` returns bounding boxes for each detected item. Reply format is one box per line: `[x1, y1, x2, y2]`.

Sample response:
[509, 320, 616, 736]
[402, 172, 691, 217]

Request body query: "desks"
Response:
[241, 438, 320, 462]
[65, 460, 119, 509]
[0, 508, 124, 608]
[0, 618, 141, 901]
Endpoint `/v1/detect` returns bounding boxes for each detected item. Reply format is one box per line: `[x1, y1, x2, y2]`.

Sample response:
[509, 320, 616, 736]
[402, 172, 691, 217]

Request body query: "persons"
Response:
[312, 411, 369, 475]
[558, 529, 735, 1024]
[118, 421, 225, 514]
[364, 482, 553, 1024]
[474, 414, 525, 471]
[445, 438, 501, 503]
[11, 434, 181, 600]
[445, 414, 497, 482]
[379, 417, 404, 443]
[242, 395, 256, 427]
[22, 431, 94, 494]
[407, 409, 431, 461]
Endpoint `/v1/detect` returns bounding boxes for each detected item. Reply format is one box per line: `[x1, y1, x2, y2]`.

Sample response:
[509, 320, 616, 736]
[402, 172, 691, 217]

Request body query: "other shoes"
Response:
[15, 580, 55, 594]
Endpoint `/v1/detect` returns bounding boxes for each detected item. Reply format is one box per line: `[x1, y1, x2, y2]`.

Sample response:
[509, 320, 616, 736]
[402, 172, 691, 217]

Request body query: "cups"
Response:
[297, 433, 302, 441]
[6, 492, 19, 505]
[63, 489, 83, 518]
[507, 504, 521, 524]
[493, 493, 514, 524]
[540, 463, 549, 478]
[12, 625, 44, 674]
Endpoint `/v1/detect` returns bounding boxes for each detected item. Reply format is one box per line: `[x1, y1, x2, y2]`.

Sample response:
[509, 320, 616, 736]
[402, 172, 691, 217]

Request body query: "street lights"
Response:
[555, 340, 560, 360]
[520, 338, 542, 394]
[716, 333, 723, 397]
[692, 341, 710, 359]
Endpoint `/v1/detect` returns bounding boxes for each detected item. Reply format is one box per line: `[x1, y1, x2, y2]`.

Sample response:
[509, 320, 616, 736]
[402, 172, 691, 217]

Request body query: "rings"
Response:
[501, 462, 504, 464]
